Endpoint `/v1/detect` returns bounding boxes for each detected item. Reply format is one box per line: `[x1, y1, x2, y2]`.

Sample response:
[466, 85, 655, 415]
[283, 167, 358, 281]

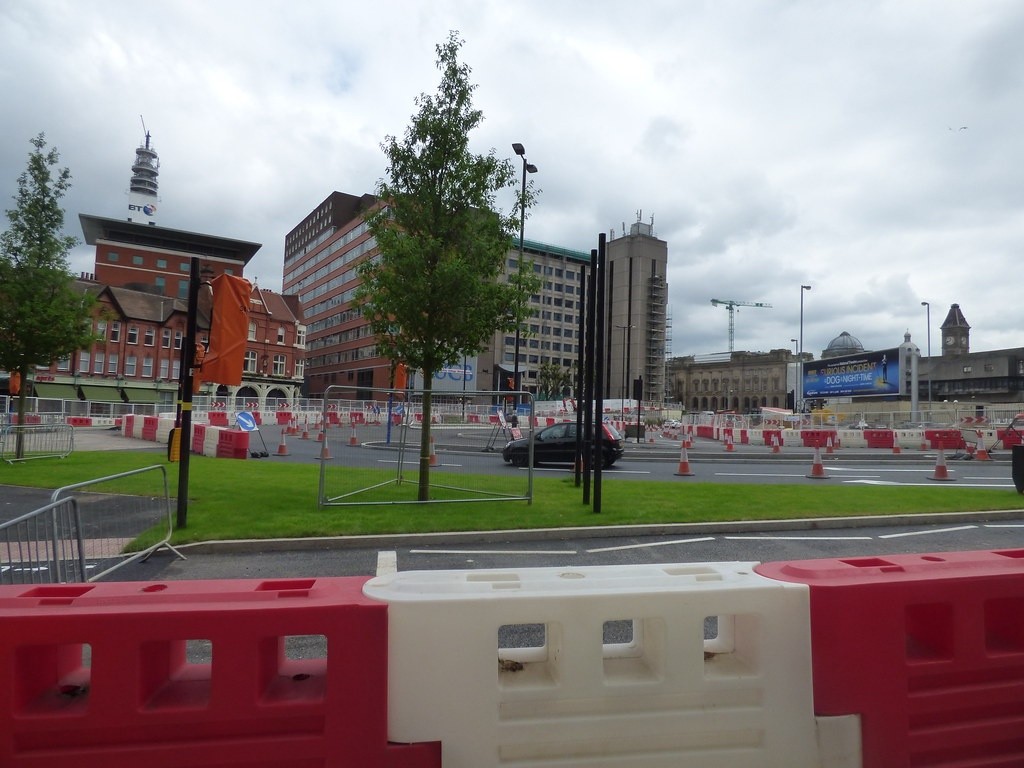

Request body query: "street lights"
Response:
[790, 339, 799, 414]
[799, 285, 812, 414]
[921, 301, 931, 422]
[510, 143, 539, 428]
[426, 435, 443, 467]
[615, 325, 635, 421]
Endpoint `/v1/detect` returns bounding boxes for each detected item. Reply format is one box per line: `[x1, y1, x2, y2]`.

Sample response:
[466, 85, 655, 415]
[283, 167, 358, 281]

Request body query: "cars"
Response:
[664, 418, 683, 428]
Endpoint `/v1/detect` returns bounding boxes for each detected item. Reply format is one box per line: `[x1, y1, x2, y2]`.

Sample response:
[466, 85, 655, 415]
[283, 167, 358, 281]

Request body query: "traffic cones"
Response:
[891, 431, 903, 454]
[345, 421, 362, 447]
[766, 428, 776, 448]
[610, 419, 679, 443]
[805, 440, 833, 479]
[272, 427, 293, 456]
[674, 439, 697, 477]
[770, 431, 782, 454]
[917, 432, 930, 451]
[680, 427, 695, 450]
[723, 428, 739, 453]
[314, 434, 335, 460]
[925, 441, 958, 481]
[974, 430, 993, 461]
[570, 448, 585, 474]
[832, 432, 844, 450]
[282, 410, 382, 443]
[824, 432, 834, 454]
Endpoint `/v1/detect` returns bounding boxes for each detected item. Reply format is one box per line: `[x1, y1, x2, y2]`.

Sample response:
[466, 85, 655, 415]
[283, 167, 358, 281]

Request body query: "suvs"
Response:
[503, 419, 626, 472]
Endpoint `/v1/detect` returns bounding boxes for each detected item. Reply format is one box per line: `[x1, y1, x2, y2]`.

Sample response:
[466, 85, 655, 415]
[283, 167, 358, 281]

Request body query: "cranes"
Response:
[710, 298, 774, 352]
